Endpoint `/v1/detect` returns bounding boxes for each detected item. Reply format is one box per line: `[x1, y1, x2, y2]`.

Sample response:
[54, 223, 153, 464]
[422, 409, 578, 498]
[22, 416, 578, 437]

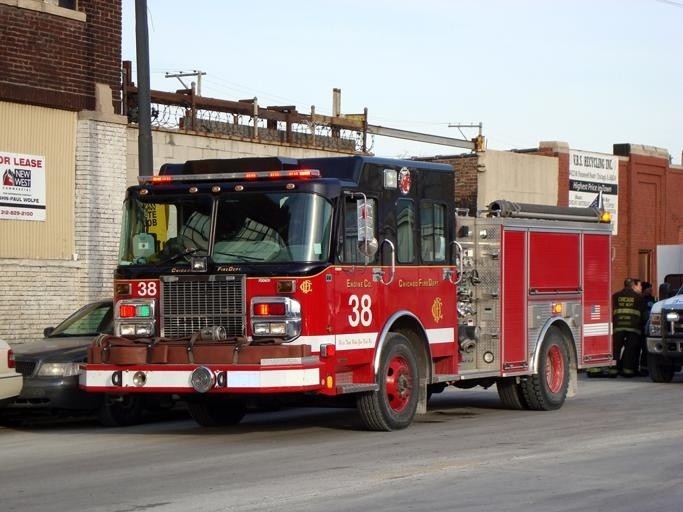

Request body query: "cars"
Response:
[0, 338, 26, 406]
[6, 296, 114, 426]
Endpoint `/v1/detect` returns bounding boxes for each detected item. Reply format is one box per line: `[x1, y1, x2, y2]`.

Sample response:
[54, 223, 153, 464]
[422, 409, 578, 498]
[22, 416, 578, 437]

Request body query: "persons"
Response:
[585, 278, 657, 378]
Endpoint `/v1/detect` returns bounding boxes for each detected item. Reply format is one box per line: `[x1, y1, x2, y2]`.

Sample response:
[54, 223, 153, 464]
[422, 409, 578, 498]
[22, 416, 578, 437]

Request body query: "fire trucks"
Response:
[82, 152, 619, 432]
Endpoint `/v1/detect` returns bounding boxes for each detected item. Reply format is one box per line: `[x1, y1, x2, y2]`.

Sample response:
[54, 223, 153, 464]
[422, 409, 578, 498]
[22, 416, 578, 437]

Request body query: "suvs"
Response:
[646, 283, 682, 383]
